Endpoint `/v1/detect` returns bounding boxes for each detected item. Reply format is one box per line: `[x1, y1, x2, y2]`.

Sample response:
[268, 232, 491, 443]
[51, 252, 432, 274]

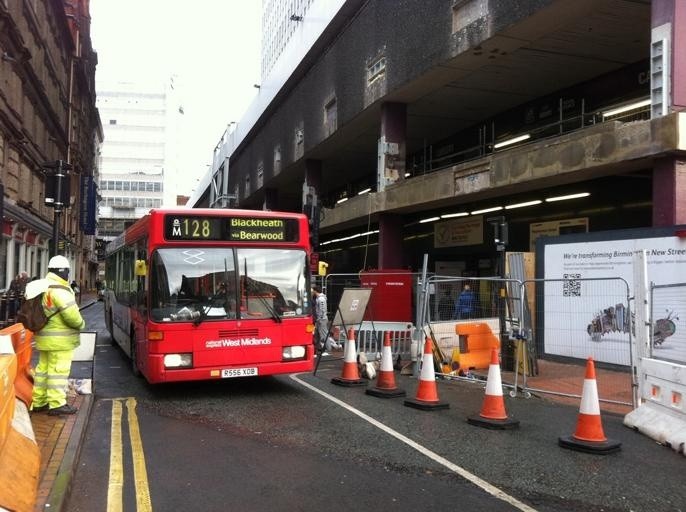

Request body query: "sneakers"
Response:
[321, 352, 332, 356]
[33, 403, 77, 415]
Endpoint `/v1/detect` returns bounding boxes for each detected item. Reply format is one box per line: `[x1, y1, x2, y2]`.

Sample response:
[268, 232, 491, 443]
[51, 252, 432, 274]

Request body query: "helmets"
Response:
[47, 255, 71, 269]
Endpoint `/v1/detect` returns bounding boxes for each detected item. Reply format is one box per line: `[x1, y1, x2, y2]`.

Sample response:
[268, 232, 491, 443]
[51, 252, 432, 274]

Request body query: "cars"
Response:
[98, 280, 105, 301]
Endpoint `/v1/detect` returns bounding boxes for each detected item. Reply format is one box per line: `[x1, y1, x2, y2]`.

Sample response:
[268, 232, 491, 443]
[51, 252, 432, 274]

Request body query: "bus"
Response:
[104, 208, 328, 384]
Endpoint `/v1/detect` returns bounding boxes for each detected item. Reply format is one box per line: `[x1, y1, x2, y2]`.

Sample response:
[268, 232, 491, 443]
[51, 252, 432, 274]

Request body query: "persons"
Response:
[18, 253, 86, 414]
[454, 283, 480, 319]
[312, 284, 331, 357]
[19, 271, 32, 286]
[438, 290, 455, 321]
[94, 278, 101, 295]
[9, 274, 23, 291]
[70, 280, 77, 291]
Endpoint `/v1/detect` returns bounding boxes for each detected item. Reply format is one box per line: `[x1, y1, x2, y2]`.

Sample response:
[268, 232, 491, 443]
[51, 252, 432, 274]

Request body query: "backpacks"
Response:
[17, 285, 71, 332]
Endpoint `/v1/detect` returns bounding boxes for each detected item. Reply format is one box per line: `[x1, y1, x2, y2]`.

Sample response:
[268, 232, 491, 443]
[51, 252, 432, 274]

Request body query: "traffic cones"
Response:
[403, 336, 451, 412]
[330, 325, 344, 355]
[467, 346, 521, 431]
[331, 327, 369, 389]
[558, 357, 623, 456]
[366, 330, 405, 400]
